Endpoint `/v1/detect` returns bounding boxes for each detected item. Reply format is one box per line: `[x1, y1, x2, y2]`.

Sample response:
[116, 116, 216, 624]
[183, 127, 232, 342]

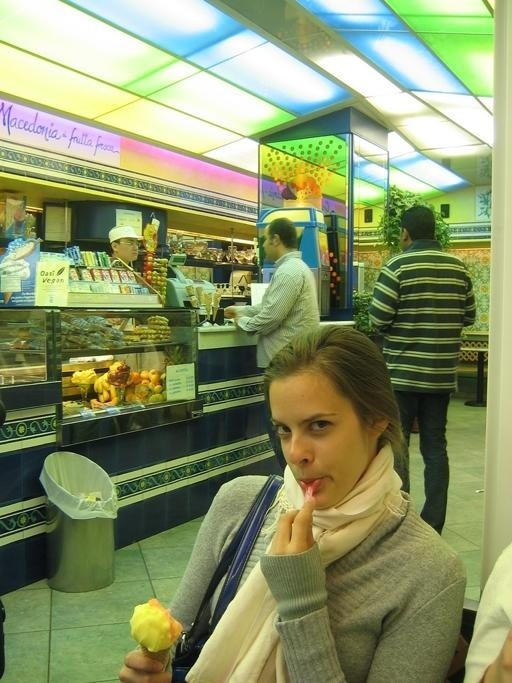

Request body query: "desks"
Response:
[461, 347, 487, 405]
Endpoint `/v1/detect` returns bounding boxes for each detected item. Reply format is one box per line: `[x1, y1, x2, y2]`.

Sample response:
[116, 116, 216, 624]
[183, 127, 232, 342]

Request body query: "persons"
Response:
[107, 225, 144, 270]
[368, 205, 477, 535]
[222, 217, 320, 470]
[118, 324, 467, 683]
[462, 542, 512, 682]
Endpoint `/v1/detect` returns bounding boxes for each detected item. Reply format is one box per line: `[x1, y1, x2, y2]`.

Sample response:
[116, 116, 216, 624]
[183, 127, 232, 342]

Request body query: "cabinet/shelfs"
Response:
[1, 307, 204, 447]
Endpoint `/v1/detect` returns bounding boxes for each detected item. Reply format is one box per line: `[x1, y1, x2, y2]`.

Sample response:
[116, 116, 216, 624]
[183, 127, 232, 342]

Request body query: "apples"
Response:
[128, 370, 166, 403]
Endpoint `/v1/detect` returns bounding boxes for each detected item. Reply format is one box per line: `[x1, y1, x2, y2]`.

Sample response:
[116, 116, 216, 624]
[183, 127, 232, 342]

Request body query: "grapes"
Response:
[107, 365, 131, 385]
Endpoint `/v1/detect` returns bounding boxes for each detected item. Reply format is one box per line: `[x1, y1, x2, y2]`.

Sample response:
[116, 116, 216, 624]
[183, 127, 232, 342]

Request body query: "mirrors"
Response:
[257, 131, 389, 310]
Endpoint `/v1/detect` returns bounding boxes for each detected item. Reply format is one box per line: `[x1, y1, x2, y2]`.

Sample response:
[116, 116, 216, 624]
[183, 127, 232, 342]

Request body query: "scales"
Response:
[166, 253, 221, 316]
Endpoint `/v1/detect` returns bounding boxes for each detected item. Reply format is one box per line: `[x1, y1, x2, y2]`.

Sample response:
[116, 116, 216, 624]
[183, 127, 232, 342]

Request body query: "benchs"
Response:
[455, 341, 487, 399]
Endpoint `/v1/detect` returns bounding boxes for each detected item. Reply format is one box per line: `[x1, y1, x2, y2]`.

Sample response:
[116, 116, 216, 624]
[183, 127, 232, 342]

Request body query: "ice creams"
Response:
[129, 596, 186, 674]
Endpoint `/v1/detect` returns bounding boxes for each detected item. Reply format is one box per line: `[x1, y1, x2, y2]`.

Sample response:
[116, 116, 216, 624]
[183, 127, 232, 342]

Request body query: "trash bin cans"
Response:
[44, 451, 117, 593]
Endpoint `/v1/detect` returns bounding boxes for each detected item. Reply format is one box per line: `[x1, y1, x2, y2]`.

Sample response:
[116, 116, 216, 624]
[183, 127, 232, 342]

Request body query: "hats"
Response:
[108, 226, 140, 243]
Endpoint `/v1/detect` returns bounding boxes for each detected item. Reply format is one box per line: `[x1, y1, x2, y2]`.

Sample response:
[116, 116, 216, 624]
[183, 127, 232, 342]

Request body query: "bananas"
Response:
[90, 363, 123, 408]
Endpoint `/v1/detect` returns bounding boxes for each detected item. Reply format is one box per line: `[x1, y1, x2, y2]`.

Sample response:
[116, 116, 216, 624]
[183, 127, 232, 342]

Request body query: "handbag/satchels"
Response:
[170, 472, 284, 682]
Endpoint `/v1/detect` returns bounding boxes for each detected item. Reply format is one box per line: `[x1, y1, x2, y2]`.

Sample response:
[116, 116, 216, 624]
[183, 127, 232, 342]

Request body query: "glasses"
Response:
[120, 241, 141, 247]
